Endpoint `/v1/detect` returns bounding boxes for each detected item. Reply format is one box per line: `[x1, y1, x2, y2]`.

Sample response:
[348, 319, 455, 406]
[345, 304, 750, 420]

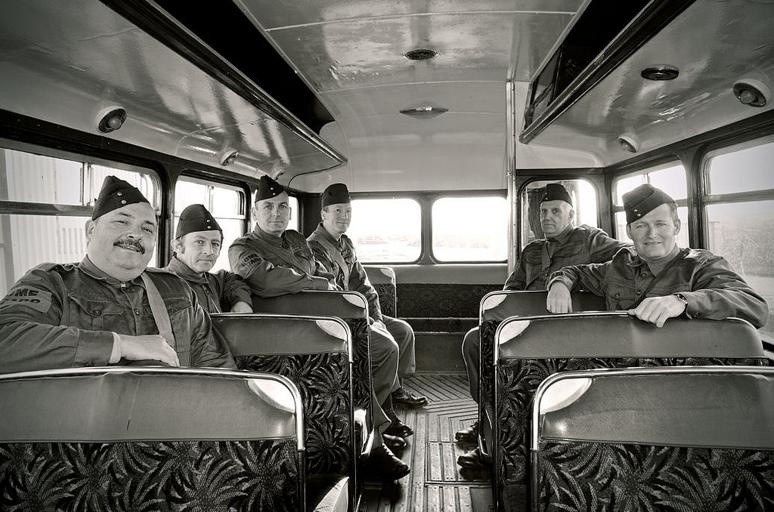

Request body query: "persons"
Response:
[305, 182, 427, 412]
[457, 183, 769, 485]
[0, 174, 239, 374]
[229, 173, 412, 454]
[146, 203, 412, 498]
[452, 182, 636, 447]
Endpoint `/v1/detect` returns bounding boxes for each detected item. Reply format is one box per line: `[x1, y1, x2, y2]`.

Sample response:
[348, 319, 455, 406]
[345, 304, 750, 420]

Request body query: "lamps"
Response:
[731, 79, 771, 109]
[617, 131, 642, 156]
[215, 147, 240, 167]
[93, 104, 128, 135]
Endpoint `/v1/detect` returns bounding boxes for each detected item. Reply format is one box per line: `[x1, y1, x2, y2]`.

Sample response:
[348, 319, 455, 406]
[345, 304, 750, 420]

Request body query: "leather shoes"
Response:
[374, 442, 411, 480]
[455, 422, 478, 443]
[383, 432, 409, 451]
[393, 386, 431, 408]
[385, 408, 414, 439]
[456, 447, 486, 471]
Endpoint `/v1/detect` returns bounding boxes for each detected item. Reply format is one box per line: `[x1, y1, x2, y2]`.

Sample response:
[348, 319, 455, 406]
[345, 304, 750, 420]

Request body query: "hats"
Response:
[321, 183, 350, 207]
[538, 183, 574, 206]
[91, 175, 149, 222]
[254, 174, 284, 204]
[620, 184, 678, 224]
[175, 203, 222, 239]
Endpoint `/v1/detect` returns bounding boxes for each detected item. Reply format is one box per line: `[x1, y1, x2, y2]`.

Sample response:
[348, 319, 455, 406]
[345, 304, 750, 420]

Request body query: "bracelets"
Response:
[674, 289, 688, 312]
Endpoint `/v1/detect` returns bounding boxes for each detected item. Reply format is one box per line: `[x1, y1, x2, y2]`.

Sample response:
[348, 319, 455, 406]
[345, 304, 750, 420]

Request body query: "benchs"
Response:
[477, 285, 773, 511]
[0, 265, 398, 512]
[391, 266, 525, 374]
